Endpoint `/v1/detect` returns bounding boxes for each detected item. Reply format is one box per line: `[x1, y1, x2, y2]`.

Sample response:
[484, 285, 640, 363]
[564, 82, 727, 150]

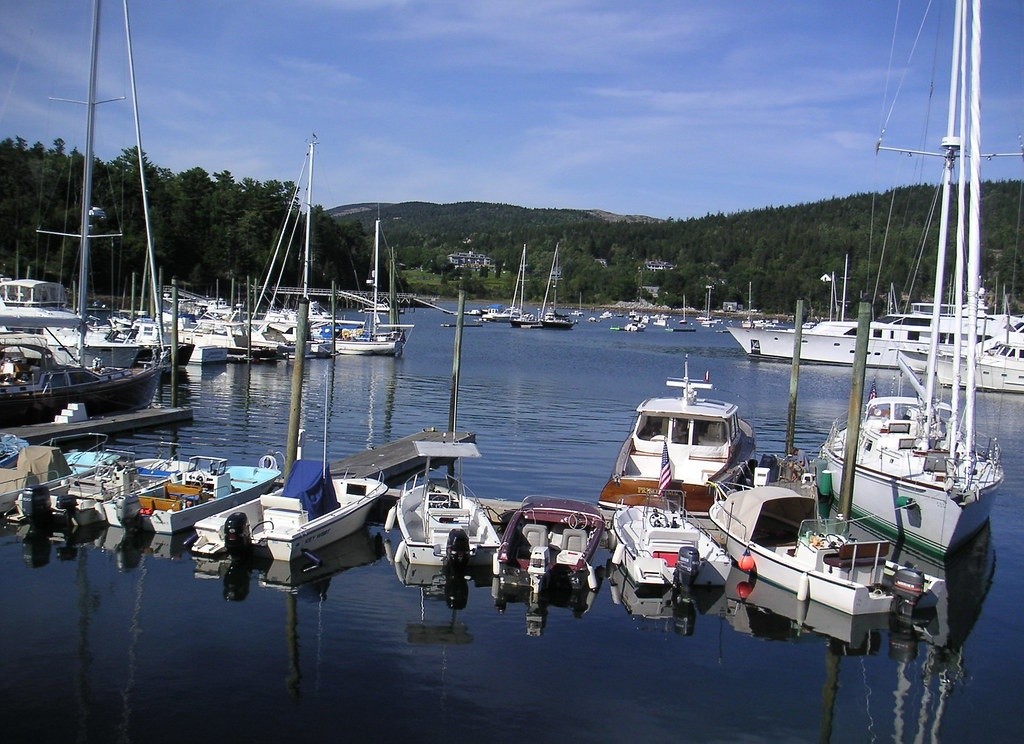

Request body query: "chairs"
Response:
[699, 424, 724, 446]
[563, 529, 586, 552]
[515, 523, 549, 559]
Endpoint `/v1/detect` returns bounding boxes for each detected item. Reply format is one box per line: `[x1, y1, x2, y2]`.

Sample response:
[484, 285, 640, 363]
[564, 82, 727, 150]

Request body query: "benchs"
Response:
[642, 526, 700, 550]
[260, 493, 308, 532]
[136, 481, 203, 511]
[823, 540, 889, 582]
[426, 508, 471, 533]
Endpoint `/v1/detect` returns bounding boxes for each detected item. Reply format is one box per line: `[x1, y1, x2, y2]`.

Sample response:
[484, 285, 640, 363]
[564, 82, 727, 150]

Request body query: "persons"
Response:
[791, 447, 811, 472]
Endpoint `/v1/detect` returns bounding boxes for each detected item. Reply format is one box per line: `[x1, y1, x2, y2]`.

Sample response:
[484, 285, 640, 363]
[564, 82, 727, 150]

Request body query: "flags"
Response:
[866, 379, 877, 417]
[658, 443, 674, 495]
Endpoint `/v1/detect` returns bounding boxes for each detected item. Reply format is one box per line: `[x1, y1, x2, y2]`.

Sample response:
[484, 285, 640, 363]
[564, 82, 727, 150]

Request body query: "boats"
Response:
[608, 492, 733, 587]
[726, 254, 989, 369]
[0, 431, 282, 533]
[493, 495, 606, 594]
[724, 553, 950, 649]
[384, 540, 493, 645]
[191, 526, 384, 684]
[709, 481, 945, 617]
[570, 309, 817, 332]
[182, 364, 389, 563]
[889, 314, 1024, 393]
[491, 578, 596, 637]
[607, 557, 728, 637]
[385, 340, 501, 566]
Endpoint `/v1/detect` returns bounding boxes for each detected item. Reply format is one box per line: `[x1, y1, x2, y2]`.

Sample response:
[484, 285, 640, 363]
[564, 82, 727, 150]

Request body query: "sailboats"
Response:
[814, 0, 1024, 566]
[481, 243, 530, 322]
[510, 242, 575, 330]
[819, 505, 996, 744]
[0, 0, 415, 423]
